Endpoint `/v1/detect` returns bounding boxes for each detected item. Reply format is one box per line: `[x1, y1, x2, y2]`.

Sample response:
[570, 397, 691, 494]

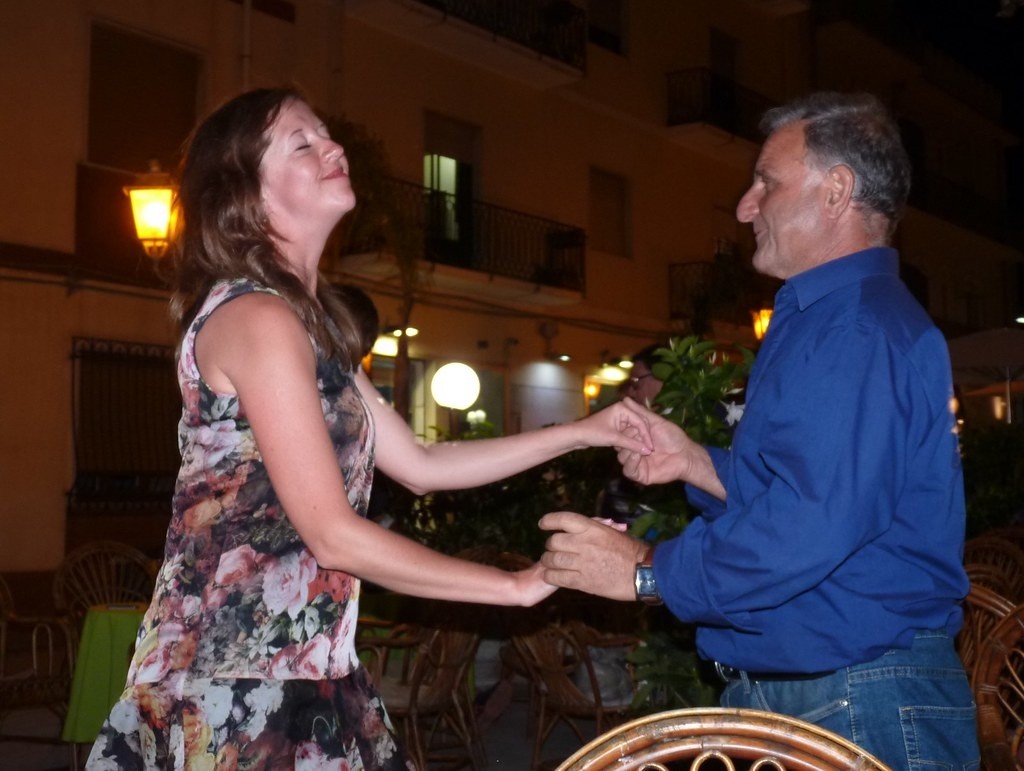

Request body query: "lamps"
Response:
[123, 156, 181, 283]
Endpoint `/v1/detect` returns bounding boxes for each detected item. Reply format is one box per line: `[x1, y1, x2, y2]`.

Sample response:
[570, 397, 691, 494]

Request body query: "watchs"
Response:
[633, 548, 663, 606]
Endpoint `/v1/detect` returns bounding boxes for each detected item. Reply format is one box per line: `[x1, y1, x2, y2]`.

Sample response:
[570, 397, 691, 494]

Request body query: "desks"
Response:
[64, 603, 476, 771]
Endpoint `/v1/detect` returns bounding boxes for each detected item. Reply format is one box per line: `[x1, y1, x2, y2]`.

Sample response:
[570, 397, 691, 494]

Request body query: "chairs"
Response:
[556, 707, 892, 771]
[381, 546, 538, 771]
[533, 228, 582, 288]
[0, 577, 81, 771]
[954, 584, 1020, 729]
[966, 562, 1010, 600]
[964, 538, 1020, 599]
[356, 545, 499, 771]
[970, 606, 1024, 771]
[53, 541, 161, 676]
[493, 551, 662, 771]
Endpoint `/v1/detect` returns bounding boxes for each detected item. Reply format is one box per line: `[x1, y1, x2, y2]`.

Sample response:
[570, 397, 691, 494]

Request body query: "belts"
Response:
[714, 661, 837, 682]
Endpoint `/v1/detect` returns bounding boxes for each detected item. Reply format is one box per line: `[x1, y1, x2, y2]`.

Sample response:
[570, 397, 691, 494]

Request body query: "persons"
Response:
[538, 91, 981, 771]
[84, 87, 653, 771]
[320, 276, 669, 534]
